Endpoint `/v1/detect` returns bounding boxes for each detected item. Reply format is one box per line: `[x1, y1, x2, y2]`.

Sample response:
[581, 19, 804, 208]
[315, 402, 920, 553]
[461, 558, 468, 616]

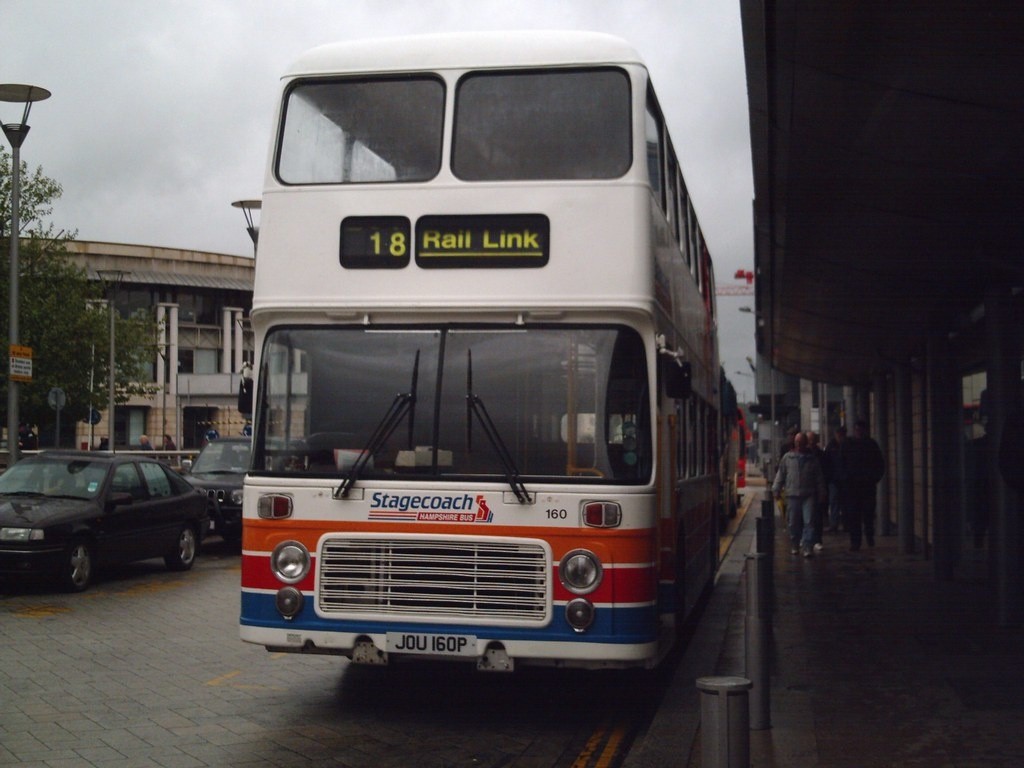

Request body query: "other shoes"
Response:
[813, 543, 823, 550]
[867, 547, 879, 560]
[791, 548, 799, 556]
[849, 548, 861, 562]
[803, 551, 813, 557]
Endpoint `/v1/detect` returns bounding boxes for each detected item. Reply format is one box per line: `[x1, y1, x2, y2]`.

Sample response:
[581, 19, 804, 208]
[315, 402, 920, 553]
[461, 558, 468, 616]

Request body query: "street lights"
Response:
[231, 199, 294, 466]
[96, 268, 133, 451]
[0, 83, 53, 469]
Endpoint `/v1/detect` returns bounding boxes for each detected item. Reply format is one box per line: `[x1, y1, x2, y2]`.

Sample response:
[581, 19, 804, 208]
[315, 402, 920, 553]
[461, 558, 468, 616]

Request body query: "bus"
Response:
[720, 379, 753, 533]
[231, 28, 722, 672]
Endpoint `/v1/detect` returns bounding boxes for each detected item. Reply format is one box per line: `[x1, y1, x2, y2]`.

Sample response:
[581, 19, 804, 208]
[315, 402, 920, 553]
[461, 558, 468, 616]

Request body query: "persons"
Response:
[163, 434, 176, 451]
[769, 418, 885, 557]
[139, 435, 153, 450]
[18, 418, 38, 451]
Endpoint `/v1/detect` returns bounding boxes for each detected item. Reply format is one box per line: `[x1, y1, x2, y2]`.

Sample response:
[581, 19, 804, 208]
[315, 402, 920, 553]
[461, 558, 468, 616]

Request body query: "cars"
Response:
[180, 435, 308, 554]
[0, 447, 212, 594]
